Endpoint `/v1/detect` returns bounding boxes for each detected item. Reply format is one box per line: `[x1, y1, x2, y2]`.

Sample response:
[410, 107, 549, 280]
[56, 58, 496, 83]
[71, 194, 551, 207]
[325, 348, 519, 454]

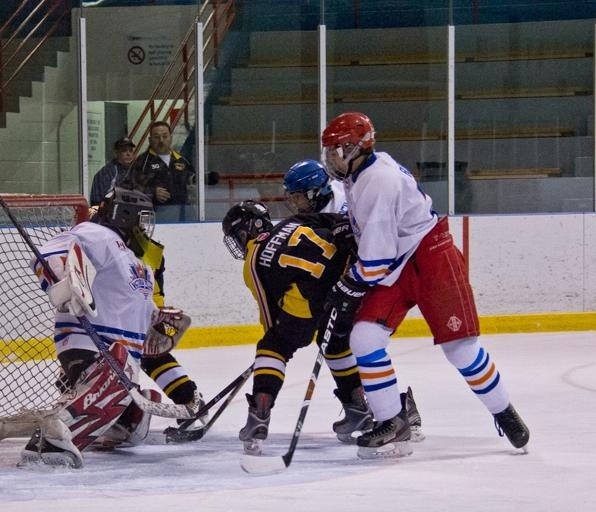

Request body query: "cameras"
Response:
[174, 168, 219, 190]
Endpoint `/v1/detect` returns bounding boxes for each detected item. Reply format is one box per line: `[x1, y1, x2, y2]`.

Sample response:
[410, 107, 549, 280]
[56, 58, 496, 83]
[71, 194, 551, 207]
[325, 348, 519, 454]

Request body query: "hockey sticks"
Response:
[239, 249, 351, 474]
[1, 198, 195, 420]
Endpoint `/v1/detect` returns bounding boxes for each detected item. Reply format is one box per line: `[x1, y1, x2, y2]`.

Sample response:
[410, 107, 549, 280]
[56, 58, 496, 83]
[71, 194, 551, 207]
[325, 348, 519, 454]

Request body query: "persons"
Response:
[89, 204, 208, 447]
[25, 190, 206, 456]
[320, 114, 530, 449]
[219, 202, 370, 438]
[133, 120, 187, 210]
[282, 162, 352, 247]
[91, 137, 136, 213]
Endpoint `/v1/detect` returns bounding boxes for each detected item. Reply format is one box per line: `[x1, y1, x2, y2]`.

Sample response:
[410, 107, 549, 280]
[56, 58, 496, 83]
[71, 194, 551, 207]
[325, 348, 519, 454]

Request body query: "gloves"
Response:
[323, 276, 369, 333]
[46, 244, 98, 320]
[143, 307, 191, 357]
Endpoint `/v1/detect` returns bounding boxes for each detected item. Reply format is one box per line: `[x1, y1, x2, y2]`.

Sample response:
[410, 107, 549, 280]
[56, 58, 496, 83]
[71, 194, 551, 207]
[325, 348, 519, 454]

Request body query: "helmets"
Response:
[322, 112, 376, 182]
[283, 160, 333, 215]
[223, 200, 273, 260]
[93, 187, 155, 257]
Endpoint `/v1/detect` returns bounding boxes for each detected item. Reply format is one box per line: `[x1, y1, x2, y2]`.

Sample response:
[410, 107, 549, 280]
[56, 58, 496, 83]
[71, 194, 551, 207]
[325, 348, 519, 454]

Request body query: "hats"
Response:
[116, 138, 135, 150]
